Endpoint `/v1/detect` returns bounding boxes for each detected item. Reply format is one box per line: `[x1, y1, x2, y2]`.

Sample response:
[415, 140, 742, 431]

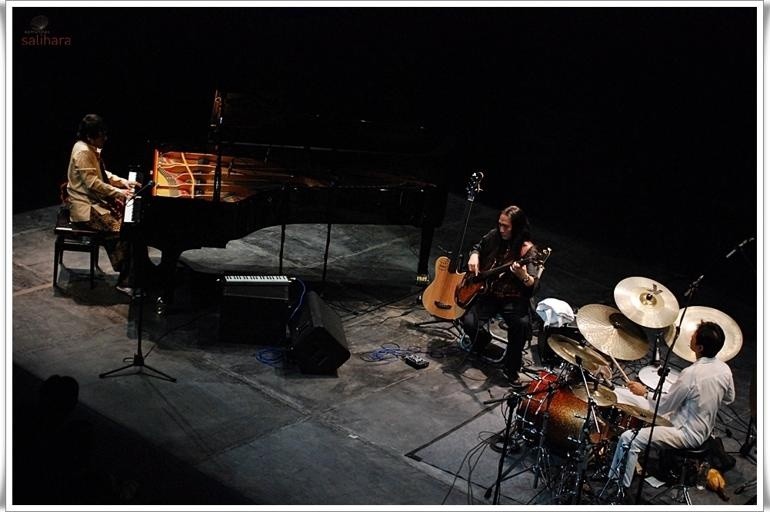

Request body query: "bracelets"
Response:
[521, 274, 530, 283]
[643, 391, 649, 400]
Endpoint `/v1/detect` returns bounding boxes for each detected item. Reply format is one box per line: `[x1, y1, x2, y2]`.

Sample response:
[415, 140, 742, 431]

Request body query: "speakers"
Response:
[289, 290, 351, 378]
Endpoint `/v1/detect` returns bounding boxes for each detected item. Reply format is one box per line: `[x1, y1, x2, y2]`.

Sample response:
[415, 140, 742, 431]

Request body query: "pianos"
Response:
[216, 270, 292, 286]
[120, 151, 447, 326]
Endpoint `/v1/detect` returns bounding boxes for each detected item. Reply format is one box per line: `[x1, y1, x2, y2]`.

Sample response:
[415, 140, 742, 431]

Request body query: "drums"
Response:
[516, 371, 610, 463]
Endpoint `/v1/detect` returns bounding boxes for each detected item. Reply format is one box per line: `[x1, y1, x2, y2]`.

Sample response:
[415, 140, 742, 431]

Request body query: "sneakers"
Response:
[116, 280, 132, 293]
[501, 366, 522, 387]
[472, 332, 492, 352]
[592, 483, 617, 499]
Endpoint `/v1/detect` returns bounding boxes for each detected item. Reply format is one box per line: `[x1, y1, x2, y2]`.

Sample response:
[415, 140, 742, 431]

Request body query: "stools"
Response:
[54, 207, 103, 287]
[650, 445, 706, 505]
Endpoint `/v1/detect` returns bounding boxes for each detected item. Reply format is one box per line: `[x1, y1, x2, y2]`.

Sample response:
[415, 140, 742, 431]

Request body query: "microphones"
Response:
[127, 179, 156, 202]
[470, 169, 486, 189]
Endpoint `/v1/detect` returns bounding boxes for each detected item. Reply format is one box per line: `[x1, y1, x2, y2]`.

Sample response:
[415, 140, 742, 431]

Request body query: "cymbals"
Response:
[664, 305, 743, 363]
[547, 333, 612, 379]
[576, 303, 649, 361]
[572, 385, 616, 407]
[614, 403, 673, 427]
[614, 276, 680, 328]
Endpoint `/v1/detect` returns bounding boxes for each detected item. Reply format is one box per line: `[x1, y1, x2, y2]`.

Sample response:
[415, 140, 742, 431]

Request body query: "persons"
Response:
[64, 111, 144, 295]
[458, 204, 542, 383]
[607, 318, 735, 505]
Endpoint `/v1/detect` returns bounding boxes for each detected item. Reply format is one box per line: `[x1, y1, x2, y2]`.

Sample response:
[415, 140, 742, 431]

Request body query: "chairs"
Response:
[462, 297, 536, 363]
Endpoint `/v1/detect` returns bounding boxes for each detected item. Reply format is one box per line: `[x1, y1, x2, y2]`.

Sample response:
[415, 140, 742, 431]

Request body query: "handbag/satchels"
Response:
[537, 298, 575, 328]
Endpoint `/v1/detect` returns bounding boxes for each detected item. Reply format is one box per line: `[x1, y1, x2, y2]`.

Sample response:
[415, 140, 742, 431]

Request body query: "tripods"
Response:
[99, 218, 178, 384]
[487, 394, 646, 505]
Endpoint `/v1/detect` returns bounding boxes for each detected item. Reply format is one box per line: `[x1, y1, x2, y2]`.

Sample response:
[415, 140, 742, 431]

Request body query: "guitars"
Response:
[422, 172, 484, 319]
[457, 248, 552, 309]
